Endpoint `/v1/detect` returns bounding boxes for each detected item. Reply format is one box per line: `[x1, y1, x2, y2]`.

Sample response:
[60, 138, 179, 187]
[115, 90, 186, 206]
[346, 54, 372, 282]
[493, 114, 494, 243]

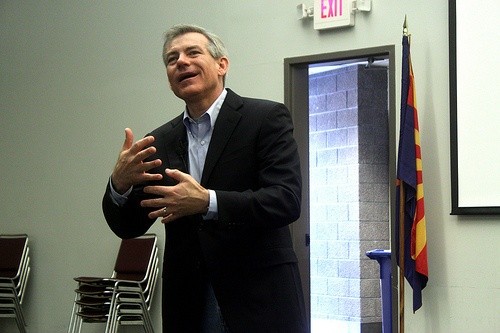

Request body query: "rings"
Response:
[164, 207, 169, 217]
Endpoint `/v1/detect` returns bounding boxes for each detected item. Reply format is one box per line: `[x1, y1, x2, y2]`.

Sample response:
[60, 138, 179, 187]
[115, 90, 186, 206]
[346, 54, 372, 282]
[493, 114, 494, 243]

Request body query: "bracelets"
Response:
[204, 190, 210, 216]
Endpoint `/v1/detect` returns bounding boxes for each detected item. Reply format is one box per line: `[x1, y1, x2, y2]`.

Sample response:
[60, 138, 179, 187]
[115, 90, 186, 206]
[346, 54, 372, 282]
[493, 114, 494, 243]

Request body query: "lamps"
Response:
[296, 0, 372, 31]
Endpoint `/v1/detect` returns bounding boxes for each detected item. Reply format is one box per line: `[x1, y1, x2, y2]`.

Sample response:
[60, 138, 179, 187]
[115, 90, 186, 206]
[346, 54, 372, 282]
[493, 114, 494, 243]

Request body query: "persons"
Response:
[102, 24, 310, 333]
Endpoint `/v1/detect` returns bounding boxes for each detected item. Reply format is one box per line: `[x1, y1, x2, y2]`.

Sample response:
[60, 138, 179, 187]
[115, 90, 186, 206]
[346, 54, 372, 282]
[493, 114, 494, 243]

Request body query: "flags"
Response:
[392, 34, 429, 314]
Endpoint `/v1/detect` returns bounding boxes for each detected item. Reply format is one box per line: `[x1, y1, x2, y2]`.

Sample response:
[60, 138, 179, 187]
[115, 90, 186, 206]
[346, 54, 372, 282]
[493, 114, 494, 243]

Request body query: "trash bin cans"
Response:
[365, 249, 392, 333]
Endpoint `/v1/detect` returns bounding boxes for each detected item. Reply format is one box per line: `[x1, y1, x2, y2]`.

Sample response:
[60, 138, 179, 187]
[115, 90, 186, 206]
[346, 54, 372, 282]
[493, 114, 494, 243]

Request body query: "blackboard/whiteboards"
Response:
[448, 1, 500, 215]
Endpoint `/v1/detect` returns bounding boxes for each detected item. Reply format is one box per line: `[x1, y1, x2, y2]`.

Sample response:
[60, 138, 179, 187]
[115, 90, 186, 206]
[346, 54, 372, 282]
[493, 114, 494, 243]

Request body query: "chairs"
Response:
[68, 233, 159, 333]
[0, 233, 31, 333]
